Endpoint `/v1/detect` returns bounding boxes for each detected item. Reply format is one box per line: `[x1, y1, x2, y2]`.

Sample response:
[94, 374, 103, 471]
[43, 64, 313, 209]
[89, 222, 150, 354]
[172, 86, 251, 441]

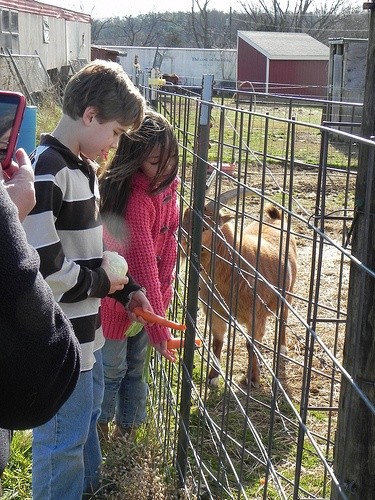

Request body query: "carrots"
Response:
[133, 307, 187, 329]
[167, 338, 201, 349]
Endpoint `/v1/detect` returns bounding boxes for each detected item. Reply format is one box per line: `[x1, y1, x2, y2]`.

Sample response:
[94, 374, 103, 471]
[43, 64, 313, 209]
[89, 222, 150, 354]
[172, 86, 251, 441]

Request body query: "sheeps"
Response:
[177, 167, 299, 391]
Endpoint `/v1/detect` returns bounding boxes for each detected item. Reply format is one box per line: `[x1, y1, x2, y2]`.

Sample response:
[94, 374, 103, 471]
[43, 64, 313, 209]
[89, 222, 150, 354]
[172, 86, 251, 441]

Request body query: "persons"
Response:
[100, 110, 178, 444]
[1, 148, 81, 473]
[0, 116, 14, 150]
[23, 59, 154, 500]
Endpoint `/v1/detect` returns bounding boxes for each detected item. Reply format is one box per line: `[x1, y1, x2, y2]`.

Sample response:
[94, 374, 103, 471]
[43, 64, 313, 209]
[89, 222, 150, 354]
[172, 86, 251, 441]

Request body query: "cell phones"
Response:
[0, 90, 26, 169]
